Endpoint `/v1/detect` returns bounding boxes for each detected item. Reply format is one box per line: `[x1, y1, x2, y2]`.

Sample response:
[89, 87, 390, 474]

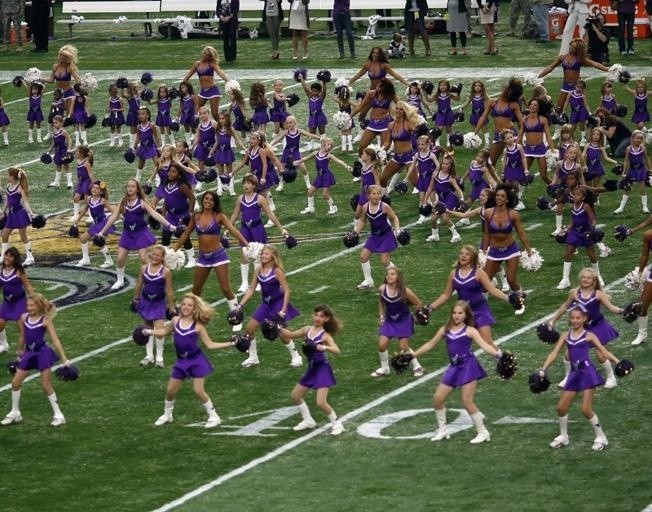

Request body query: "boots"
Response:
[470, 410, 491, 444]
[431, 407, 450, 441]
[632, 316, 648, 346]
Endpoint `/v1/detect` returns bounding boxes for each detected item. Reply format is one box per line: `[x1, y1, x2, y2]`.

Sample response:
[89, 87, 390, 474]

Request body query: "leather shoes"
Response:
[272, 51, 357, 60]
[407, 48, 499, 57]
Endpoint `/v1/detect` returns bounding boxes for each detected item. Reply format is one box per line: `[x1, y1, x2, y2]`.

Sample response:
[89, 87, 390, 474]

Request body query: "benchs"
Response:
[56, 18, 158, 36]
[316, 16, 482, 35]
[158, 17, 316, 40]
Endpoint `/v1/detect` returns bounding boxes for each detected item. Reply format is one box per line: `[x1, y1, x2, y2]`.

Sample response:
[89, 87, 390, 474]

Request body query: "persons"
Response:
[270, 305, 344, 436]
[1, 293, 73, 426]
[404, 299, 512, 444]
[508, 1, 651, 65]
[130, 244, 177, 368]
[370, 269, 424, 377]
[142, 291, 248, 428]
[1, 247, 35, 354]
[546, 267, 626, 389]
[428, 243, 524, 364]
[539, 304, 622, 451]
[1, 1, 56, 55]
[229, 244, 303, 368]
[1, 38, 652, 346]
[195, 1, 501, 58]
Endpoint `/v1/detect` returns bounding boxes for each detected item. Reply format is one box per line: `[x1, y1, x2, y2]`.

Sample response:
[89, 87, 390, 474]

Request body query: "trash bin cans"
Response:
[25, 0, 56, 40]
[549, 12, 568, 40]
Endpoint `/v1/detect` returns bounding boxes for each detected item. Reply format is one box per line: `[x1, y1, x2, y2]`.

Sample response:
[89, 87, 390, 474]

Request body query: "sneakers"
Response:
[100, 260, 113, 268]
[0, 411, 23, 425]
[227, 283, 261, 332]
[370, 367, 391, 377]
[290, 356, 304, 368]
[140, 356, 154, 366]
[204, 415, 222, 428]
[50, 414, 66, 426]
[604, 377, 619, 389]
[0, 343, 10, 353]
[67, 182, 72, 189]
[156, 360, 164, 368]
[22, 257, 34, 266]
[413, 366, 423, 377]
[241, 355, 259, 369]
[128, 144, 236, 210]
[263, 183, 338, 228]
[341, 144, 354, 152]
[579, 140, 587, 146]
[76, 258, 91, 266]
[514, 298, 526, 315]
[549, 198, 649, 290]
[549, 434, 570, 448]
[184, 261, 195, 268]
[154, 415, 173, 426]
[414, 196, 526, 242]
[331, 425, 344, 435]
[592, 438, 609, 451]
[0, 255, 4, 264]
[357, 279, 375, 289]
[111, 280, 123, 290]
[430, 134, 492, 150]
[4, 138, 124, 148]
[501, 282, 510, 292]
[47, 181, 60, 187]
[559, 377, 567, 388]
[292, 419, 316, 431]
